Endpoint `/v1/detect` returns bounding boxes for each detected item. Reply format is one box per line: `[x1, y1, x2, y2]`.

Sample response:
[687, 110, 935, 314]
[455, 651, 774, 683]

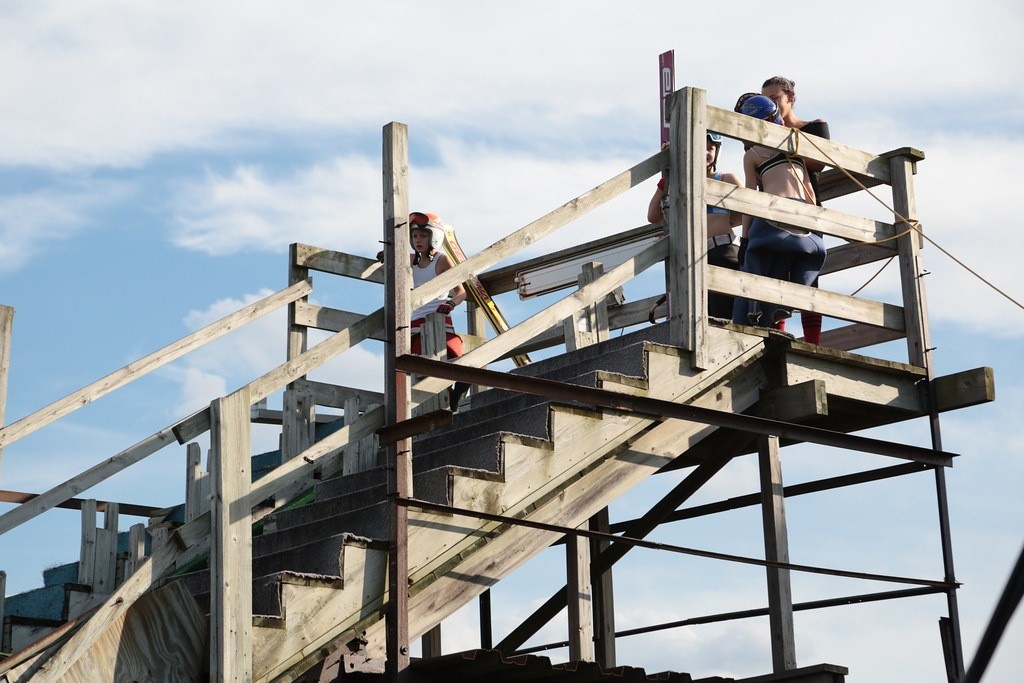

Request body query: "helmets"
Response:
[739, 95, 780, 124]
[408, 212, 445, 249]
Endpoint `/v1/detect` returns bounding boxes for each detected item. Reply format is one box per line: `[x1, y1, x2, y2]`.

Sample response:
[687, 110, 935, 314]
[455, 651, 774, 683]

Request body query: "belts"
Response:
[707, 234, 742, 250]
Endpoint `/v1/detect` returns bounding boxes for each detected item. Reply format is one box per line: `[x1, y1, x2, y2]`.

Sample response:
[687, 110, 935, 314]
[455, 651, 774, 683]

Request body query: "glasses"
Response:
[734, 93, 758, 113]
[707, 131, 722, 142]
[409, 212, 429, 227]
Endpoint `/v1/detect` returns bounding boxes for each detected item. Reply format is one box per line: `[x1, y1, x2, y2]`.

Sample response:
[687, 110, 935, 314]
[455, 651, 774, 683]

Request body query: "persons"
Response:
[647, 131, 745, 330]
[730, 93, 827, 331]
[760, 78, 831, 345]
[376, 212, 472, 411]
